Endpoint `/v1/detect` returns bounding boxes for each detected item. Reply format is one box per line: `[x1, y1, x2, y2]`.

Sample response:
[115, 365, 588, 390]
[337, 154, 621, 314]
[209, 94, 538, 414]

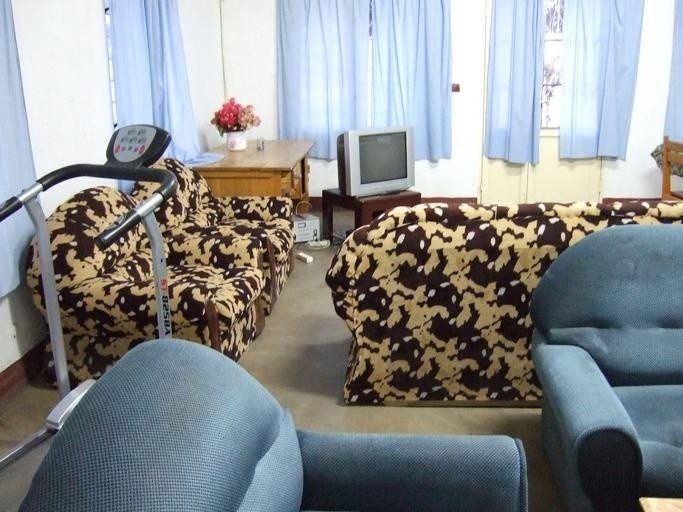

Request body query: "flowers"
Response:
[211, 96, 262, 137]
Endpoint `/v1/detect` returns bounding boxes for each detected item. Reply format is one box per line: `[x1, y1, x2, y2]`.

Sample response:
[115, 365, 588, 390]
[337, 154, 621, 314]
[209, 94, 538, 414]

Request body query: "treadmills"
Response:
[0, 124, 178, 511]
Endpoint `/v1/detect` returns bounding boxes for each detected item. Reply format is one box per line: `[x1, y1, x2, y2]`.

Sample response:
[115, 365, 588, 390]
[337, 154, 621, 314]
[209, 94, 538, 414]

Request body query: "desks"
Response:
[188, 138, 315, 217]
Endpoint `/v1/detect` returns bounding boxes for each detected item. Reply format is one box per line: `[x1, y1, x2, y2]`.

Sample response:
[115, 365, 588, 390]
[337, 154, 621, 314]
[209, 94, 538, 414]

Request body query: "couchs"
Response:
[327, 201, 683, 406]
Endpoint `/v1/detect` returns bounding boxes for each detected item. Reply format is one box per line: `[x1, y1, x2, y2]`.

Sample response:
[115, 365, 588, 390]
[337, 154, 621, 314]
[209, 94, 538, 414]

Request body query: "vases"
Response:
[226, 131, 247, 150]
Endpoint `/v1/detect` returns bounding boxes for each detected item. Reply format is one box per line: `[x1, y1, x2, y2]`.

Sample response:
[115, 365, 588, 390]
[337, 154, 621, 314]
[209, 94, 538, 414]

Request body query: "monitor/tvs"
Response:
[337, 127, 415, 197]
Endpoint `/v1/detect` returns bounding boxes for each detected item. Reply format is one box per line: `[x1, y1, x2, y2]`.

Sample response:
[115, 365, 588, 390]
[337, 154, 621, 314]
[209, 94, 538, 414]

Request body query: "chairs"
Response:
[19, 337, 530, 511]
[24, 185, 264, 388]
[662, 135, 683, 202]
[130, 156, 296, 315]
[529, 223, 682, 511]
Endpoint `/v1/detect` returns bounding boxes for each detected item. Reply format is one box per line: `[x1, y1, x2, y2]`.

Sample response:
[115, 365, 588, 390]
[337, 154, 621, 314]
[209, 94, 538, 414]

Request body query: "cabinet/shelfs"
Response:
[322, 188, 422, 243]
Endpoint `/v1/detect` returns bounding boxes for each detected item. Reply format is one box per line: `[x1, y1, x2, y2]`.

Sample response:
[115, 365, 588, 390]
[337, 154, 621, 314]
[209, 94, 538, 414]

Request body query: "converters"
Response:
[297, 252, 314, 263]
